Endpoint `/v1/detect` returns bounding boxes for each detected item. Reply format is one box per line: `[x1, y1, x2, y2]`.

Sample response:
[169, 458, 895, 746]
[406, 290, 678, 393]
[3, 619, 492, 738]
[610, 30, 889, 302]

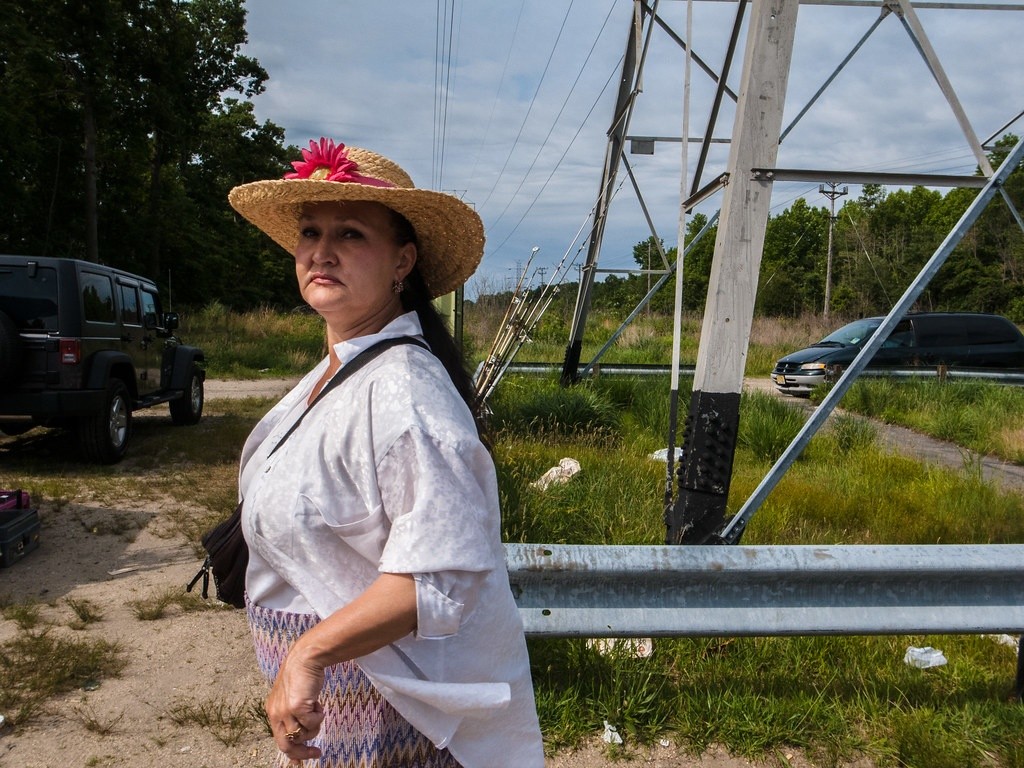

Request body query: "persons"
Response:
[230, 127, 546, 768]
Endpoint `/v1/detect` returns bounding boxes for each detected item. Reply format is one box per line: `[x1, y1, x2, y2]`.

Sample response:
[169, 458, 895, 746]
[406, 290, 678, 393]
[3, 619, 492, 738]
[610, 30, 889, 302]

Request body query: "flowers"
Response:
[282, 136, 360, 183]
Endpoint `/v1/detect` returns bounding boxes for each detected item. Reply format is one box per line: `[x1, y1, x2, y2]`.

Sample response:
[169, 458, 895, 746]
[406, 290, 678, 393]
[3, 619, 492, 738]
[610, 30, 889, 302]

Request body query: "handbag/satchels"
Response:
[186, 499, 249, 609]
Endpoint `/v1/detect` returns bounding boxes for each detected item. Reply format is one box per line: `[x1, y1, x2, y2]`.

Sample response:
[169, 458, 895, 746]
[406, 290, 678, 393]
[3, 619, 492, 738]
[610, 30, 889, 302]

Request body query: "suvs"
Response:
[0, 254, 207, 466]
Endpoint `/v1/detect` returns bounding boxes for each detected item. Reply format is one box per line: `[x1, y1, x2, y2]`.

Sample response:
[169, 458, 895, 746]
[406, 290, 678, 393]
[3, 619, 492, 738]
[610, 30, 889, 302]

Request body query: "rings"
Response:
[283, 725, 302, 741]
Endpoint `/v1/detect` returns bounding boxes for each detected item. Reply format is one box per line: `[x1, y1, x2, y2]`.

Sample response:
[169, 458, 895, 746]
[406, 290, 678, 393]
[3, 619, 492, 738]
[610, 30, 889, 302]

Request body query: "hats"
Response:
[228, 137, 486, 300]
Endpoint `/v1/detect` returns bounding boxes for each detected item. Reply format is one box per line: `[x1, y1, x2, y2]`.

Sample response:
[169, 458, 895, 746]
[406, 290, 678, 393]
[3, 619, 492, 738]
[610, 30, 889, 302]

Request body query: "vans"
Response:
[770, 311, 1024, 397]
